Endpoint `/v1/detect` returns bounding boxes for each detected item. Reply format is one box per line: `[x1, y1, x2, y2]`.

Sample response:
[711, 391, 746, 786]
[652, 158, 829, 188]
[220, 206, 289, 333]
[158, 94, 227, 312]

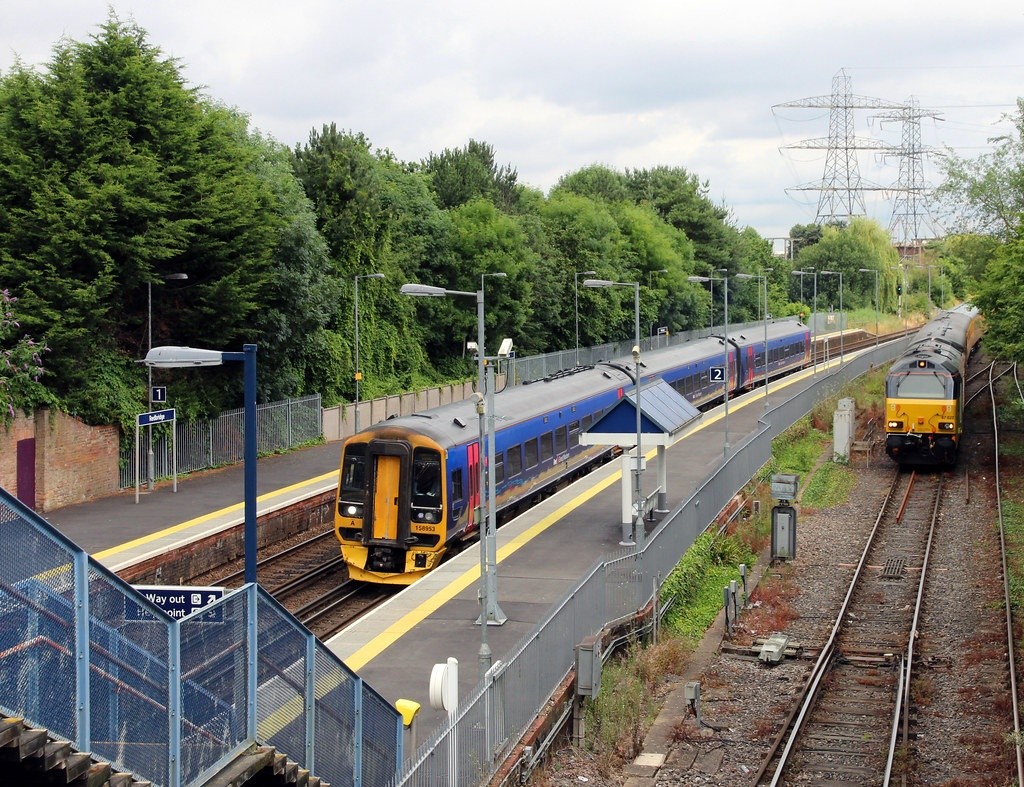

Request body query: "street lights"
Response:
[352, 271, 385, 433]
[687, 274, 730, 457]
[800, 266, 816, 312]
[397, 280, 506, 627]
[574, 269, 598, 367]
[820, 268, 846, 368]
[857, 268, 879, 349]
[649, 270, 669, 291]
[757, 267, 773, 327]
[479, 269, 509, 294]
[790, 270, 817, 378]
[138, 341, 261, 584]
[145, 273, 189, 490]
[734, 272, 769, 408]
[708, 267, 729, 335]
[579, 276, 645, 524]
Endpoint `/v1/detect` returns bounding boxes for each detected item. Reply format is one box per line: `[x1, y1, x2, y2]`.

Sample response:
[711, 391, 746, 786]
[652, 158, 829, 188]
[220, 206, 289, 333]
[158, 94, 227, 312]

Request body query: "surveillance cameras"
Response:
[499, 338, 513, 358]
[467, 342, 479, 356]
[632, 346, 639, 357]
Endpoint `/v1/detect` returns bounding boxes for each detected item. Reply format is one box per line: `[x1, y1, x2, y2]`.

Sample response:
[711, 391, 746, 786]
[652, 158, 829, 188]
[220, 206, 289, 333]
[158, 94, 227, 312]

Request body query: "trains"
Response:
[332, 317, 815, 592]
[882, 295, 1002, 473]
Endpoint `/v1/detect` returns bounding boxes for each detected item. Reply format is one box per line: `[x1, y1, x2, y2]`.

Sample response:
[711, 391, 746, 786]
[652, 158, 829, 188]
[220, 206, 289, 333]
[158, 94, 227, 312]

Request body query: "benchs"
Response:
[631, 486, 661, 522]
